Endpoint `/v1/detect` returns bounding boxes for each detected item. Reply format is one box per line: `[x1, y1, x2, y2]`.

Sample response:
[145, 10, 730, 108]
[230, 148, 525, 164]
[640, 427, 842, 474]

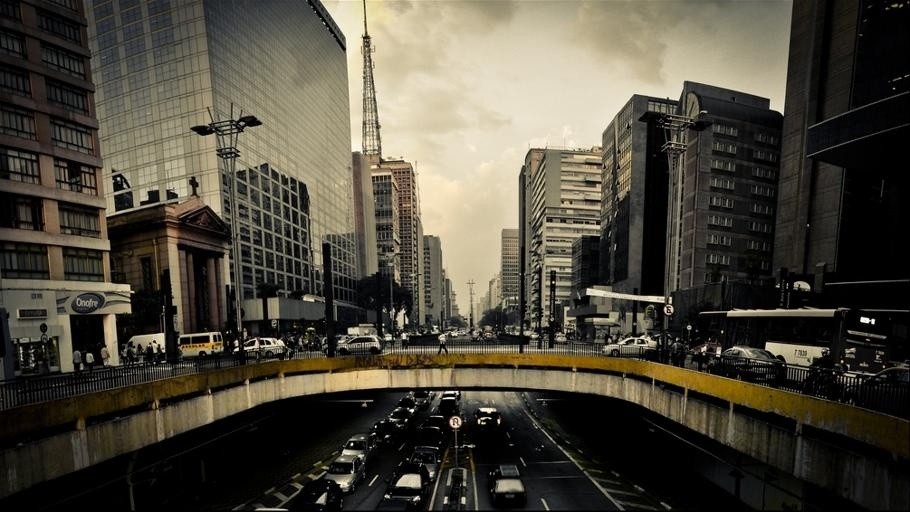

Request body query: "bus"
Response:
[721, 309, 890, 388]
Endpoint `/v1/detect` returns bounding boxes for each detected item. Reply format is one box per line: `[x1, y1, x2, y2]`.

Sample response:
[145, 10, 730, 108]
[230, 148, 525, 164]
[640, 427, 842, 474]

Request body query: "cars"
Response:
[377, 448, 439, 511]
[369, 389, 435, 446]
[488, 464, 527, 507]
[445, 327, 466, 338]
[323, 334, 383, 356]
[708, 348, 777, 386]
[289, 434, 375, 511]
[841, 367, 909, 416]
[554, 333, 566, 344]
[232, 337, 286, 358]
[601, 336, 658, 356]
[473, 408, 501, 428]
[416, 389, 460, 448]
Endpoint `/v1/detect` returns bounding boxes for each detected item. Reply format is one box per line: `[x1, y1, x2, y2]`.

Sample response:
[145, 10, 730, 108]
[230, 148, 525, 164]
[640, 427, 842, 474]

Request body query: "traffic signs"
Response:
[449, 417, 461, 429]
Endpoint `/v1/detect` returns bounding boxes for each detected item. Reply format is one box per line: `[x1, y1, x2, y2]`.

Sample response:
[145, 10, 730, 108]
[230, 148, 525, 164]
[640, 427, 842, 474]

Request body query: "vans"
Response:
[125, 333, 166, 356]
[178, 331, 224, 359]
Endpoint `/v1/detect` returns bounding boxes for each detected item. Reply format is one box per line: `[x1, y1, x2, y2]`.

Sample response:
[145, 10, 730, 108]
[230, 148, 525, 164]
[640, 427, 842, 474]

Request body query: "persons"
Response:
[223, 327, 324, 360]
[400, 330, 409, 349]
[604, 332, 715, 367]
[808, 347, 837, 398]
[71, 336, 164, 378]
[435, 332, 450, 354]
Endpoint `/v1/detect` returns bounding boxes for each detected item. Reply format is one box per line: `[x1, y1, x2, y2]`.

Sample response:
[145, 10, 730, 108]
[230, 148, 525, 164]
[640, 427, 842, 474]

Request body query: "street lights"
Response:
[378, 250, 403, 327]
[191, 104, 262, 333]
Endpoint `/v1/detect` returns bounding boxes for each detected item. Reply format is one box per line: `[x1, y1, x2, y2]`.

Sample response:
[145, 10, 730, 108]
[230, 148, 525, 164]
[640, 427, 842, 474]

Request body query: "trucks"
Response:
[348, 327, 395, 345]
